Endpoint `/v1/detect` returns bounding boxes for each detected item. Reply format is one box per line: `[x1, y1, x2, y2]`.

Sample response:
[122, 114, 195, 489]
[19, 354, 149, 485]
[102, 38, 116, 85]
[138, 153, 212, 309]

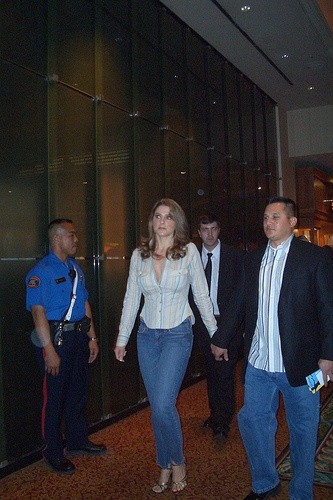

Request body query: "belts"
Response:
[61, 322, 76, 332]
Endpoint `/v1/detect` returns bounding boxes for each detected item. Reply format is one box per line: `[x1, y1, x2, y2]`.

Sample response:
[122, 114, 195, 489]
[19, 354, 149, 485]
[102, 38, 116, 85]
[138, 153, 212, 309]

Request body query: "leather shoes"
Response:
[44, 454, 75, 475]
[65, 439, 106, 456]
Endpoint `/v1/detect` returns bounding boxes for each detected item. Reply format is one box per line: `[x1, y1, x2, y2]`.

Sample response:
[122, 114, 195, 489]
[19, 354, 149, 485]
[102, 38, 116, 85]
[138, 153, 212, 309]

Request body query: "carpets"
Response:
[274, 389, 333, 488]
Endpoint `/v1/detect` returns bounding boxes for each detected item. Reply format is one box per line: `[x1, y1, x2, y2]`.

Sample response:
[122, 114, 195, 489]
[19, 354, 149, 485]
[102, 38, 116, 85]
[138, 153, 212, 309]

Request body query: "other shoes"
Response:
[212, 424, 230, 436]
[203, 410, 217, 429]
[242, 482, 281, 500]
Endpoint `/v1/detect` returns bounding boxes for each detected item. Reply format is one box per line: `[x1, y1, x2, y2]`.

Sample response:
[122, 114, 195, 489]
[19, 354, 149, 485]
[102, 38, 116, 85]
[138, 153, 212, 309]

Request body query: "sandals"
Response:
[152, 462, 172, 494]
[170, 460, 188, 494]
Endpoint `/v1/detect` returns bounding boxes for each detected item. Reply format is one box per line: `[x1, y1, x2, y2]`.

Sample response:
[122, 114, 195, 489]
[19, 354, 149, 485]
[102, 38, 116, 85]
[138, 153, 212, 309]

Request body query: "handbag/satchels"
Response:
[23, 322, 60, 359]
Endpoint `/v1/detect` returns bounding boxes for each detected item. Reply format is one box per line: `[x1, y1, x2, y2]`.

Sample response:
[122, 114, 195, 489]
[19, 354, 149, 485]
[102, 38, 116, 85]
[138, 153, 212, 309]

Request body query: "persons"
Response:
[209, 197, 333, 500]
[113, 199, 218, 493]
[24, 218, 107, 476]
[187, 214, 244, 441]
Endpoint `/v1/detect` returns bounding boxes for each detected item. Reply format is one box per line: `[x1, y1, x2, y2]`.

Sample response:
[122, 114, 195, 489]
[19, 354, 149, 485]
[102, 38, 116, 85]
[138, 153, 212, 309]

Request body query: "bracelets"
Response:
[89, 337, 97, 342]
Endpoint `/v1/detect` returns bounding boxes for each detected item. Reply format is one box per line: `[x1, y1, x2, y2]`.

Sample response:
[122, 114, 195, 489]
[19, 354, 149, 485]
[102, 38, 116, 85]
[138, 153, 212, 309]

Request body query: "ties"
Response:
[203, 253, 213, 298]
